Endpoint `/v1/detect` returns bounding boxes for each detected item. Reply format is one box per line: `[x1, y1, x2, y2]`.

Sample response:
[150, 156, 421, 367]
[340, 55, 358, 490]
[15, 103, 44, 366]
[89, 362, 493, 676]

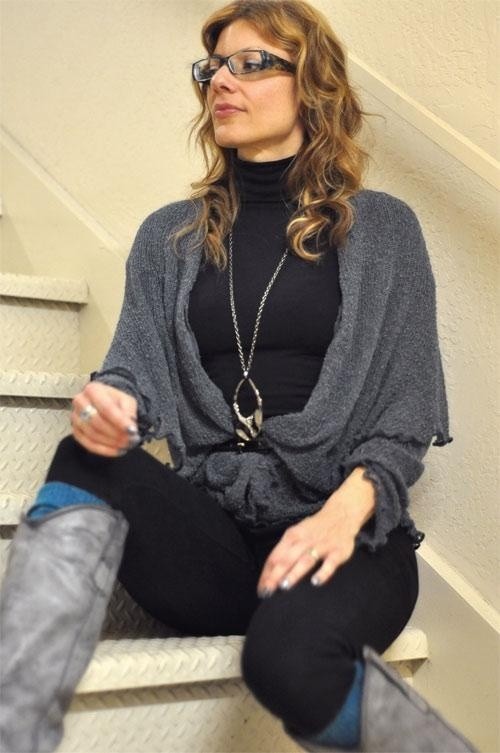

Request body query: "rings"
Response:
[309, 548, 319, 561]
[81, 405, 98, 419]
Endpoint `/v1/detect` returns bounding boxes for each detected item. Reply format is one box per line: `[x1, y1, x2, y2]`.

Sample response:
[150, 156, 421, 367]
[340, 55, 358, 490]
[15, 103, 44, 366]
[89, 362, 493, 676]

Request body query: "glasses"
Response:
[191, 48, 297, 85]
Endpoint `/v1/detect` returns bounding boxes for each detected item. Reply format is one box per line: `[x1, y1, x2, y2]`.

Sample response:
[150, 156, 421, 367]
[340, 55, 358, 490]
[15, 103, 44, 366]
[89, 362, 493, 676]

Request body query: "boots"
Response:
[286, 645, 476, 752]
[0, 493, 130, 753]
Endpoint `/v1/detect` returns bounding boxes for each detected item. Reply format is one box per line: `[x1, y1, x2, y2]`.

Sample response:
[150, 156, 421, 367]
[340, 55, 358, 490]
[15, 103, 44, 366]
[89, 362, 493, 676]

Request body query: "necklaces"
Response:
[219, 228, 290, 450]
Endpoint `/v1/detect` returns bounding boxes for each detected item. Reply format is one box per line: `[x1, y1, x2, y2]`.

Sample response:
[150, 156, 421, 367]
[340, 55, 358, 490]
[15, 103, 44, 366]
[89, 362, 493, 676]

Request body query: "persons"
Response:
[1, 0, 485, 752]
